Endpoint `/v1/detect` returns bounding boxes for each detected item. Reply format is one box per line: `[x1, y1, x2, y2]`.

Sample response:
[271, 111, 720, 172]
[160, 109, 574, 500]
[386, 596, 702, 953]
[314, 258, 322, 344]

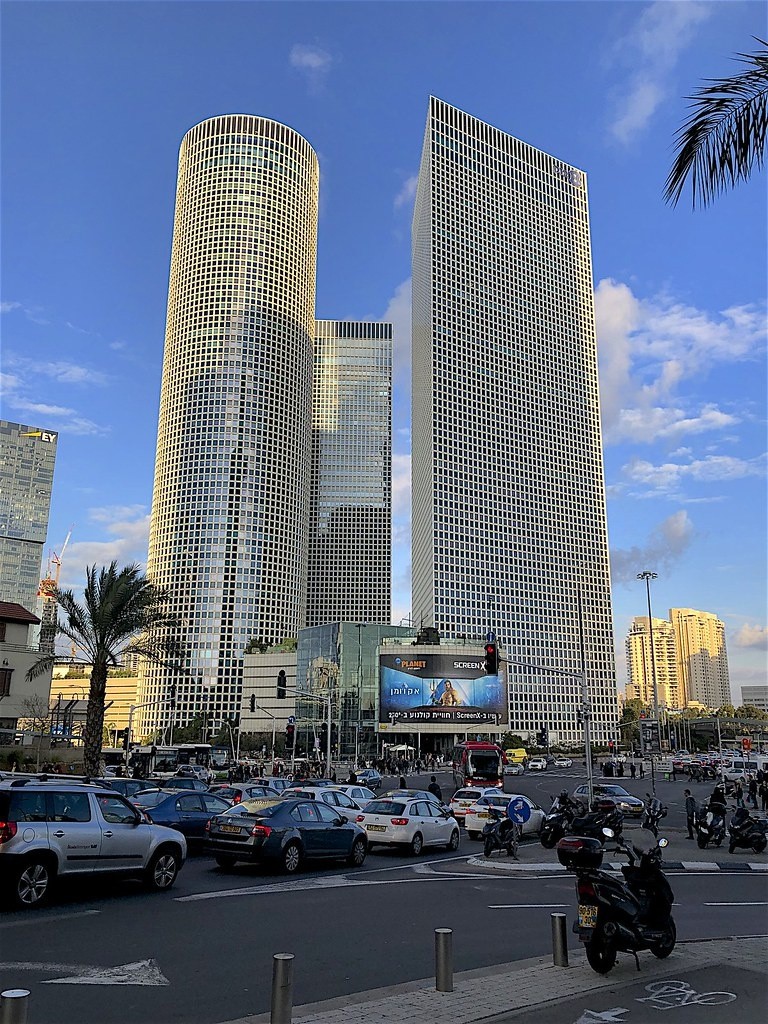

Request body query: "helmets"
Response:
[561, 789, 569, 796]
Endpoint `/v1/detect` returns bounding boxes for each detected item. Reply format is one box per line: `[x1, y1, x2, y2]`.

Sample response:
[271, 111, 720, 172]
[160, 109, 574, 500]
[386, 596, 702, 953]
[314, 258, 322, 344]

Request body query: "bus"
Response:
[101, 746, 179, 779]
[453, 740, 506, 790]
[170, 743, 231, 782]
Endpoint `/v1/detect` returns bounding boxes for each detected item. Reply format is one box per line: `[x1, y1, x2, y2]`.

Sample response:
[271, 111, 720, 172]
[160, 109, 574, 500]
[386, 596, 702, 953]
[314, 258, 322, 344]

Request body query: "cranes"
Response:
[52, 522, 74, 584]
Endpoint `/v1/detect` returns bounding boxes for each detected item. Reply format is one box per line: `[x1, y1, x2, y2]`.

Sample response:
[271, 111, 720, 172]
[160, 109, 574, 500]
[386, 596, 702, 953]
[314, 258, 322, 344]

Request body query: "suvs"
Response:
[0, 772, 187, 909]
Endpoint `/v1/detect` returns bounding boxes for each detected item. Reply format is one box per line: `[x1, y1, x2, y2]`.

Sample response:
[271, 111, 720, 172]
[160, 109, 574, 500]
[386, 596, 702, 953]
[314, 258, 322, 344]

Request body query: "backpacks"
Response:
[688, 797, 696, 812]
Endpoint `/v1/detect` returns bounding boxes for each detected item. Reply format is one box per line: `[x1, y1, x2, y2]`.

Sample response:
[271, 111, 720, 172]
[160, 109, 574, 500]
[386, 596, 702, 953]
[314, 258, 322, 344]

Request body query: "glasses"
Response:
[684, 793, 687, 794]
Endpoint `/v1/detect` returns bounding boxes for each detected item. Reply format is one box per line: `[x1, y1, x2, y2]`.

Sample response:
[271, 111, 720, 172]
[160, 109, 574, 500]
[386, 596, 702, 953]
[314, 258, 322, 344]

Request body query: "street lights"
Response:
[355, 624, 366, 752]
[636, 571, 658, 714]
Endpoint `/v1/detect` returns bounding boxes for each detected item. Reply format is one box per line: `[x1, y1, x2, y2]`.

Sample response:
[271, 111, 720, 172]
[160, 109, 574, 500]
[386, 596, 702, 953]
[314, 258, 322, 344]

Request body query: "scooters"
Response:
[557, 827, 677, 973]
[480, 798, 522, 857]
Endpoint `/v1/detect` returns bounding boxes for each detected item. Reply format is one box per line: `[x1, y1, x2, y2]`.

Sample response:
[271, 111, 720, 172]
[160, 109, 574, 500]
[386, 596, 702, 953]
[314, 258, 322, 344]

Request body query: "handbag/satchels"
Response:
[746, 795, 751, 803]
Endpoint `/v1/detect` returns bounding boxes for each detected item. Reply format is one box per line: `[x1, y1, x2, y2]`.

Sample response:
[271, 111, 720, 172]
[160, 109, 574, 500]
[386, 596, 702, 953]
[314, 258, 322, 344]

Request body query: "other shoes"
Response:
[685, 834, 693, 840]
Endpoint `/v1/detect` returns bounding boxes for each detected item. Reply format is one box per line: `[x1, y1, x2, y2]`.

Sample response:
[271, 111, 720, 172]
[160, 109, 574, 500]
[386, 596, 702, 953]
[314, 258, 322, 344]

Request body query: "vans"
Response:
[720, 756, 768, 773]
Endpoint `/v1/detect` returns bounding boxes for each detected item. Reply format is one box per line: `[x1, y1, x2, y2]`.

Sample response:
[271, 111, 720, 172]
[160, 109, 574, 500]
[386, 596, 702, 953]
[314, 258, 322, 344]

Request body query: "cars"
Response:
[123, 758, 571, 875]
[573, 784, 645, 818]
[612, 748, 768, 781]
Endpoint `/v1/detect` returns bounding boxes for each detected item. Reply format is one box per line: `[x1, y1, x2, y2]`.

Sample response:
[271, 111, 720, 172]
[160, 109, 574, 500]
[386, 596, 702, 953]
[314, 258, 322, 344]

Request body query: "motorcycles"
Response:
[641, 792, 668, 837]
[693, 802, 768, 854]
[541, 789, 625, 849]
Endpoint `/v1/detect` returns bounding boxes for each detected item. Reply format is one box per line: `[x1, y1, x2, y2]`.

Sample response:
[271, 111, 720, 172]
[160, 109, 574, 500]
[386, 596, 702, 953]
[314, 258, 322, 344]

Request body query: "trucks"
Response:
[505, 748, 528, 763]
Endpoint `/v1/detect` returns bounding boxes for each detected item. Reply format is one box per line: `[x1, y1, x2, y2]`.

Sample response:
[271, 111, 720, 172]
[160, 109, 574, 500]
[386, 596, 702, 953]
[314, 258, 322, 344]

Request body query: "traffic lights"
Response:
[249, 694, 255, 712]
[609, 742, 613, 753]
[285, 723, 296, 749]
[320, 723, 327, 752]
[484, 642, 498, 676]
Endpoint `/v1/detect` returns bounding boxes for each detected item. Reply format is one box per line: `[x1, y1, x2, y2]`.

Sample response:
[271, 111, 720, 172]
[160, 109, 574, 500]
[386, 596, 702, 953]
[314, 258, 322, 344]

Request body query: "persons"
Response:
[733, 765, 768, 811]
[427, 776, 442, 801]
[348, 770, 357, 783]
[523, 756, 527, 767]
[399, 777, 408, 789]
[29, 761, 62, 773]
[408, 752, 445, 774]
[272, 762, 287, 776]
[357, 755, 409, 776]
[223, 760, 266, 784]
[630, 763, 646, 779]
[557, 789, 579, 821]
[116, 765, 129, 777]
[684, 789, 696, 840]
[688, 765, 709, 783]
[710, 787, 727, 838]
[295, 760, 336, 783]
